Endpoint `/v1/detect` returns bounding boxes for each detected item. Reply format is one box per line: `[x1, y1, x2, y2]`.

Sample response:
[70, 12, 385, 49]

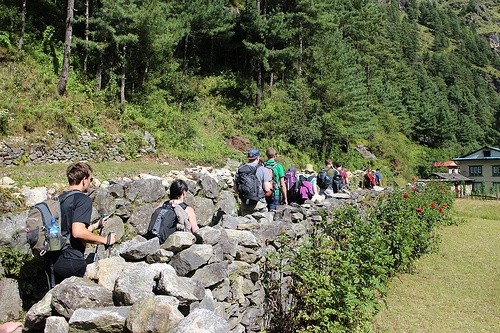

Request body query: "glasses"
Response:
[88, 177, 93, 182]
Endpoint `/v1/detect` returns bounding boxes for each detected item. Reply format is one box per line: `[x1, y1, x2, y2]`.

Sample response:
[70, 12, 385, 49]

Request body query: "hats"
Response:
[246, 149, 260, 160]
[287, 167, 296, 174]
[300, 164, 317, 174]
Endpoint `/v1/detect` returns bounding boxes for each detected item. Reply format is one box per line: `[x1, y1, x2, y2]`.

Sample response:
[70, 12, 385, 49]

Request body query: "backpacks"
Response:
[236, 163, 263, 202]
[284, 171, 297, 203]
[147, 200, 190, 245]
[25, 191, 88, 289]
[333, 171, 343, 193]
[297, 175, 317, 203]
[317, 167, 331, 189]
[261, 162, 279, 201]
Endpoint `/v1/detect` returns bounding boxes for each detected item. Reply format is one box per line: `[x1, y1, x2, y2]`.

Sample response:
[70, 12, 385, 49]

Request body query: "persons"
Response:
[163, 179, 200, 234]
[51, 162, 117, 284]
[234, 147, 382, 216]
[0, 321, 24, 333]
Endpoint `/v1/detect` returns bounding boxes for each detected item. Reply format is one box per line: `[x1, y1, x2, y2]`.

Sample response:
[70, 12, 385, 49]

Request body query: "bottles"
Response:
[47, 218, 58, 239]
